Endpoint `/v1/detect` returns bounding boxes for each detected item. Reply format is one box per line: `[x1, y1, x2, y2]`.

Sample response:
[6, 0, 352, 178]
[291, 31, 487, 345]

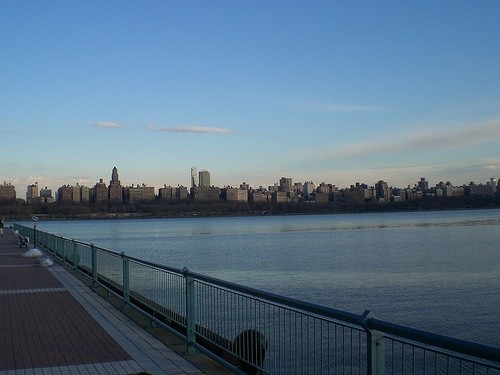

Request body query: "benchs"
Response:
[18, 234, 29, 249]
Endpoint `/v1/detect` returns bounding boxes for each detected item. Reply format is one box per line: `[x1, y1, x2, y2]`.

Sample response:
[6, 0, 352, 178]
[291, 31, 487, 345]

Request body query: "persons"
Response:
[0, 219, 4, 236]
